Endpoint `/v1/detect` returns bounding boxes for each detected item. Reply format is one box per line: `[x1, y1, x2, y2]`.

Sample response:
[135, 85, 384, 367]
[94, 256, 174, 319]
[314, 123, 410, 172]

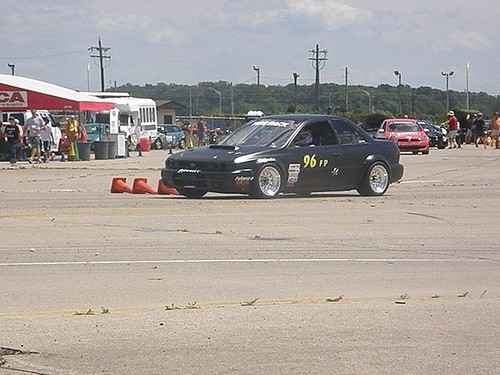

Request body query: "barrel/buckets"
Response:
[93, 141, 109, 159]
[78, 142, 91, 161]
[108, 141, 116, 159]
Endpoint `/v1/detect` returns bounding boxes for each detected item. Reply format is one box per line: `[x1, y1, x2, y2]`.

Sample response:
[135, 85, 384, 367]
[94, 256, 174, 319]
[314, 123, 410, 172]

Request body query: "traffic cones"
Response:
[110, 178, 134, 194]
[158, 178, 181, 195]
[131, 177, 158, 195]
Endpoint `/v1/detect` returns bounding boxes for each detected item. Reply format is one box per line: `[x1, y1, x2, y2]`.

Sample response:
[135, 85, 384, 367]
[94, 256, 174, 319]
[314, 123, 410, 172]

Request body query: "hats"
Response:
[42, 117, 50, 124]
[447, 110, 454, 116]
[478, 113, 482, 116]
[494, 111, 499, 115]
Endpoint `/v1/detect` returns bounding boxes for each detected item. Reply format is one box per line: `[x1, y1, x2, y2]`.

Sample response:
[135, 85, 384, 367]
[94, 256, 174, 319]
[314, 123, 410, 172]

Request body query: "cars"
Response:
[377, 119, 430, 156]
[83, 123, 111, 154]
[418, 120, 449, 150]
[152, 124, 183, 150]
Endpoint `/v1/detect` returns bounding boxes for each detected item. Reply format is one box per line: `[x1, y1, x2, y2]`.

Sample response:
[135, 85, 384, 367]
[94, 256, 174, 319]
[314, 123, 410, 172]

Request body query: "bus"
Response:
[94, 96, 158, 149]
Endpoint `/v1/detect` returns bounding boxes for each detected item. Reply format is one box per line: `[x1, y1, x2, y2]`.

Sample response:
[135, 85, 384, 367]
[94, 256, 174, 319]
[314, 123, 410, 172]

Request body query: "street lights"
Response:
[252, 65, 260, 109]
[204, 86, 222, 115]
[7, 64, 15, 76]
[393, 71, 404, 115]
[291, 72, 301, 112]
[441, 70, 454, 110]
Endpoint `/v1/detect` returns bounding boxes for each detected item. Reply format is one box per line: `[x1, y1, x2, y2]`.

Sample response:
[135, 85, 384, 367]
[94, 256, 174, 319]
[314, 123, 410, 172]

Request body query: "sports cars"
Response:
[160, 114, 404, 198]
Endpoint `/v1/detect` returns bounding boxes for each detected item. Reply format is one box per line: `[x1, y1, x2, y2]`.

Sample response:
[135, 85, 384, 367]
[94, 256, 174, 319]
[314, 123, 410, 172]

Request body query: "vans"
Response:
[0, 109, 52, 134]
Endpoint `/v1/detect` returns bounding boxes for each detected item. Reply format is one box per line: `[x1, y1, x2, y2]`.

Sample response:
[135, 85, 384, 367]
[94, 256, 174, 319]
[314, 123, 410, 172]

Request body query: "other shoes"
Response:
[27, 157, 33, 164]
[450, 145, 452, 148]
[453, 144, 457, 148]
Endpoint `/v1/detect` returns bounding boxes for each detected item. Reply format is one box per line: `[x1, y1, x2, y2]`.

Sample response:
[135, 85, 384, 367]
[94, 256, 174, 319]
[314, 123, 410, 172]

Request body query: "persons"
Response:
[444, 111, 500, 150]
[184, 115, 217, 149]
[274, 128, 314, 147]
[0, 112, 89, 166]
